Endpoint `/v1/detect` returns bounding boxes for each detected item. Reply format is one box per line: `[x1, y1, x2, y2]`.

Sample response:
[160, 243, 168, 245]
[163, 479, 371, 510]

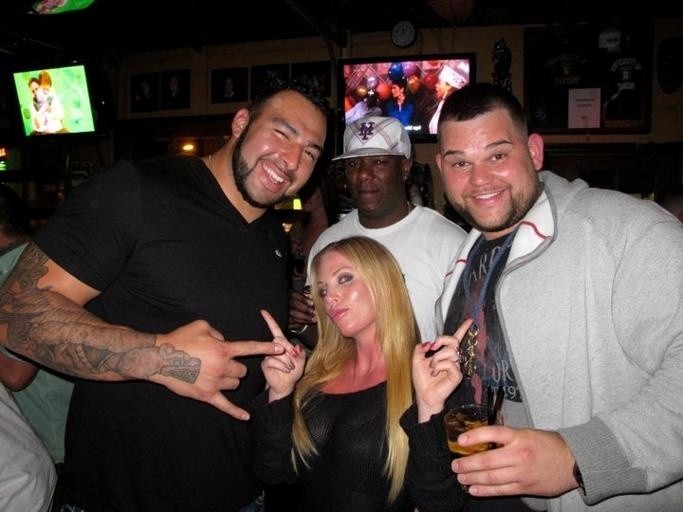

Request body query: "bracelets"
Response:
[289, 324, 309, 336]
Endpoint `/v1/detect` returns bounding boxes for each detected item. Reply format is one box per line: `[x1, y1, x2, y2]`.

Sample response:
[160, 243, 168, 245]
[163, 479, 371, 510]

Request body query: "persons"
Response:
[284, 171, 341, 292]
[288, 114, 470, 368]
[0, 385, 57, 512]
[426, 82, 683, 512]
[345, 62, 468, 132]
[259, 234, 473, 512]
[32, 71, 56, 115]
[140, 76, 319, 109]
[26, 78, 71, 136]
[0, 183, 77, 466]
[0, 70, 335, 511]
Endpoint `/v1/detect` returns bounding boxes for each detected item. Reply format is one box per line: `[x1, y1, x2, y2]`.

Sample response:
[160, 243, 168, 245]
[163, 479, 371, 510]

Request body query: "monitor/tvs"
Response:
[336, 50, 477, 144]
[12, 64, 96, 138]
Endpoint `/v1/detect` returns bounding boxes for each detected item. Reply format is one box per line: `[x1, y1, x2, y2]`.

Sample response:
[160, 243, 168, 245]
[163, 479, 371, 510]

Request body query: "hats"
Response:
[439, 66, 467, 89]
[332, 117, 412, 160]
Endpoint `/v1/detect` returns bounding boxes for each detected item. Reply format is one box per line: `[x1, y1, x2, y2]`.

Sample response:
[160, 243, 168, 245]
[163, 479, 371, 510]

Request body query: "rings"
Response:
[452, 345, 460, 363]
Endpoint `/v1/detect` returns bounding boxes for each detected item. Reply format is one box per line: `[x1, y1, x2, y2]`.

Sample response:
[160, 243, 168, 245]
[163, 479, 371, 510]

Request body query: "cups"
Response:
[443, 404, 503, 494]
